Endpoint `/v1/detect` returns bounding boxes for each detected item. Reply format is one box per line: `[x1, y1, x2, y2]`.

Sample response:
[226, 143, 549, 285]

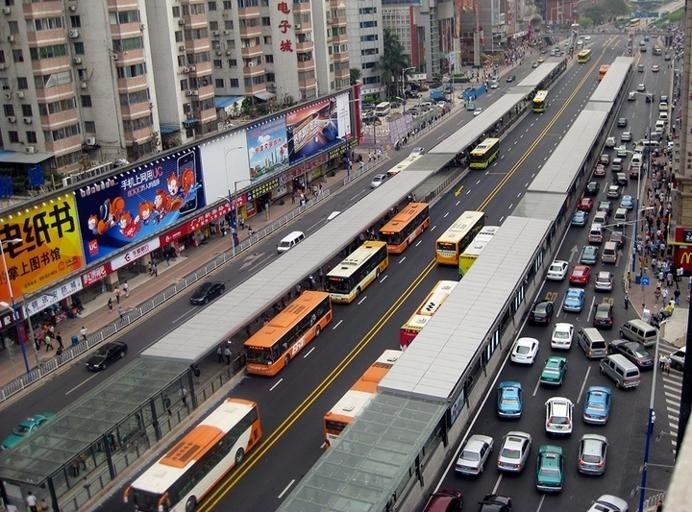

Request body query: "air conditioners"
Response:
[178, 18, 198, 96]
[83, 136, 95, 146]
[69, 6, 75, 11]
[71, 57, 81, 64]
[113, 54, 118, 60]
[213, 31, 231, 58]
[6, 116, 16, 123]
[68, 29, 79, 39]
[294, 24, 301, 29]
[140, 24, 146, 29]
[271, 83, 277, 88]
[7, 35, 15, 42]
[24, 147, 34, 153]
[23, 116, 32, 124]
[0, 64, 5, 70]
[3, 93, 12, 100]
[16, 91, 25, 98]
[2, 7, 11, 14]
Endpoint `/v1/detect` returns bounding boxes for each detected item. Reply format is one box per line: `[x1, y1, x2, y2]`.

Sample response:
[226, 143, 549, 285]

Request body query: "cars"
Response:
[325, 210, 341, 223]
[0, 409, 56, 453]
[187, 280, 228, 307]
[362, 83, 427, 190]
[82, 340, 128, 373]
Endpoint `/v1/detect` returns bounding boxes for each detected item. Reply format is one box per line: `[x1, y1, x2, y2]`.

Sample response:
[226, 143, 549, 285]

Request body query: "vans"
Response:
[276, 229, 306, 255]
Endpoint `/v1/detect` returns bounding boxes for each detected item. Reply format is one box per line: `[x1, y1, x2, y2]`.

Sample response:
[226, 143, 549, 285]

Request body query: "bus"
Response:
[325, 239, 391, 305]
[378, 200, 431, 256]
[318, 347, 402, 453]
[121, 397, 263, 512]
[241, 289, 334, 378]
[396, 279, 458, 354]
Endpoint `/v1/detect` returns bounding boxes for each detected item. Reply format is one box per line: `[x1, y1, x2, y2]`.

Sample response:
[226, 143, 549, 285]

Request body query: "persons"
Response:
[26, 66, 498, 512]
[624, 32, 686, 432]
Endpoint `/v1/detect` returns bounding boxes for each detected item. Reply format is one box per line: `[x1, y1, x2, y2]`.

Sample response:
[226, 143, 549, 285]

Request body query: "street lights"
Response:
[215, 143, 245, 260]
[0, 237, 34, 383]
[402, 64, 416, 114]
[333, 135, 350, 181]
[20, 288, 59, 367]
[233, 178, 257, 231]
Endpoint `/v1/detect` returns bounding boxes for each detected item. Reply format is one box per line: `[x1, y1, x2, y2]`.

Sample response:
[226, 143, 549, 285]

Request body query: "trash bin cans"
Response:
[71, 336, 79, 346]
[71, 464, 79, 478]
[636, 276, 641, 284]
